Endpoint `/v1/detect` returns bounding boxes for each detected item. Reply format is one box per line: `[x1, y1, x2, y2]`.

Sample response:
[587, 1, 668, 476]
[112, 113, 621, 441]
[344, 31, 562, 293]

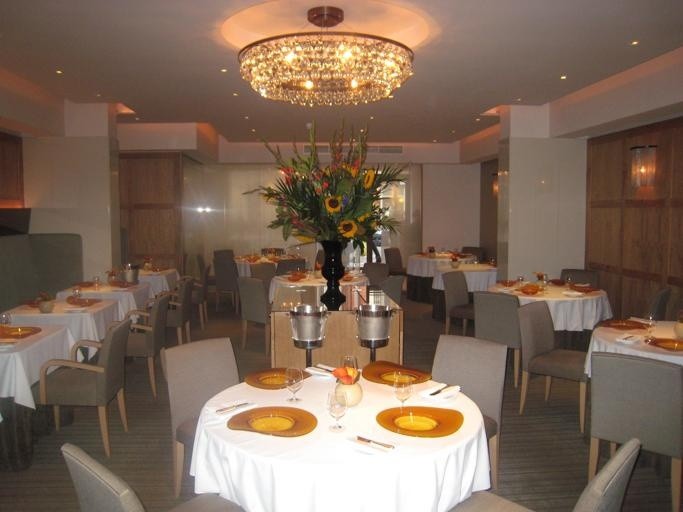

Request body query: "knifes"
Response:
[216, 402, 248, 415]
[357, 434, 396, 449]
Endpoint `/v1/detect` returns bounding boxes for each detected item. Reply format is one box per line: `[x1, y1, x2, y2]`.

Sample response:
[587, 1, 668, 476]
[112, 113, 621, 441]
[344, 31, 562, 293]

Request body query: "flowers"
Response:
[243, 117, 411, 241]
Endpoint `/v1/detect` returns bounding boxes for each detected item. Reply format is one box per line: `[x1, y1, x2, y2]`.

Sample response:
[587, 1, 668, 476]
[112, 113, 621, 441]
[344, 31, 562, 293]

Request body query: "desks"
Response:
[271, 285, 404, 368]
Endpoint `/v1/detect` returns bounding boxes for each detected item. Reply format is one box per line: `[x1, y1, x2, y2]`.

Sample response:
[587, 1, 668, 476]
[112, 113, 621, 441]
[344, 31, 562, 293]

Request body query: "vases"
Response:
[321, 241, 347, 311]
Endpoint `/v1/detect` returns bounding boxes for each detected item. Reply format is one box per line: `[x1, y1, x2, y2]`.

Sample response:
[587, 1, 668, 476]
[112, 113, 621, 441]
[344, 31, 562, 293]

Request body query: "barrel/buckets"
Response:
[122, 264, 139, 284]
[289, 304, 328, 340]
[355, 304, 390, 339]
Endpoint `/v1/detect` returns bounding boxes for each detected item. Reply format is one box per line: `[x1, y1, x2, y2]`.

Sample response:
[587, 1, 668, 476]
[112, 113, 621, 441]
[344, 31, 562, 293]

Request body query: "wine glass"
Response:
[284, 354, 415, 431]
[644, 316, 655, 342]
[93, 275, 99, 288]
[0, 314, 11, 328]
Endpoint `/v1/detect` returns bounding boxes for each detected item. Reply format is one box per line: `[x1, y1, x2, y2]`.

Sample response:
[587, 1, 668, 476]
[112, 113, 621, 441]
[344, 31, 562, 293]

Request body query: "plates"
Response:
[607, 320, 645, 331]
[1, 327, 41, 339]
[496, 278, 599, 298]
[25, 281, 128, 307]
[651, 338, 682, 351]
[287, 270, 353, 282]
[236, 255, 297, 262]
[227, 360, 464, 438]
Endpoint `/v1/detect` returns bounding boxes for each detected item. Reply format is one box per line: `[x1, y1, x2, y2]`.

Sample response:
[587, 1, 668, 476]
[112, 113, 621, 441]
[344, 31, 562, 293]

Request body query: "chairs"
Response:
[313, 249, 325, 270]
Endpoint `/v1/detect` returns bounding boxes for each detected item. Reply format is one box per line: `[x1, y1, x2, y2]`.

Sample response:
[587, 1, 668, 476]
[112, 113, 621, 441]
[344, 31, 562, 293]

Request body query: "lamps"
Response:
[238, 5, 415, 108]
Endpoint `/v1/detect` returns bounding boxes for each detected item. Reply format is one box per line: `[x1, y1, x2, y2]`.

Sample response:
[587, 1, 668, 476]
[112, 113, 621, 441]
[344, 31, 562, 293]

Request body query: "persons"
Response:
[367, 234, 381, 264]
